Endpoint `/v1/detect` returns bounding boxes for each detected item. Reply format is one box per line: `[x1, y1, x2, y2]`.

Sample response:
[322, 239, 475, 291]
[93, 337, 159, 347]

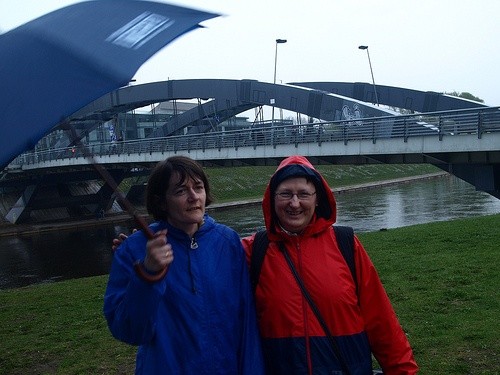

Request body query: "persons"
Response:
[112, 154, 420, 375]
[103, 156, 265, 375]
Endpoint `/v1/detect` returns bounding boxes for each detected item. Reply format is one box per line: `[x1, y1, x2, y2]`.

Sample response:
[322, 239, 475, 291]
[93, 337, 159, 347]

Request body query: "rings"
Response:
[165, 252, 169, 257]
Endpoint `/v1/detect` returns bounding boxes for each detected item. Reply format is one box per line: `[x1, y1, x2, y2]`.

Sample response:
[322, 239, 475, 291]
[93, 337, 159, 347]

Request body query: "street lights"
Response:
[359, 45, 379, 107]
[270, 39, 287, 145]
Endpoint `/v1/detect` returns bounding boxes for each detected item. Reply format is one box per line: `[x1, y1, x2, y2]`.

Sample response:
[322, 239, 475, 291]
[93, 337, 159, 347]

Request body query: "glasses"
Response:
[274, 191, 317, 200]
